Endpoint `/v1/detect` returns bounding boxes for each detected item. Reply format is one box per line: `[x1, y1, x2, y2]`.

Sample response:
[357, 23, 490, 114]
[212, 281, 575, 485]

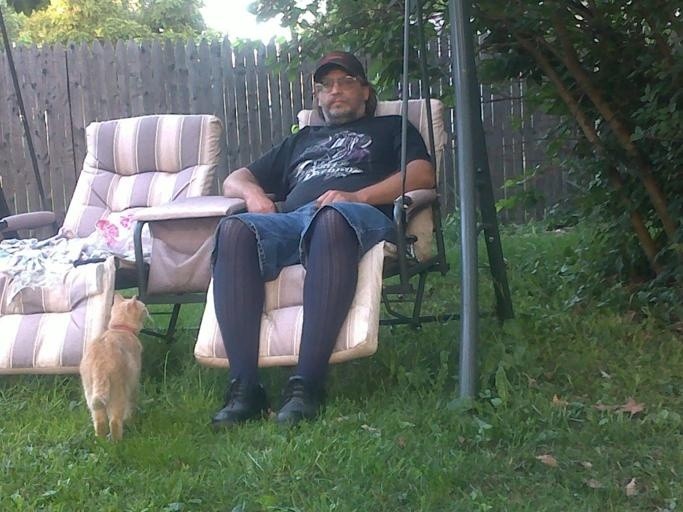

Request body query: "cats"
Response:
[78, 293, 150, 444]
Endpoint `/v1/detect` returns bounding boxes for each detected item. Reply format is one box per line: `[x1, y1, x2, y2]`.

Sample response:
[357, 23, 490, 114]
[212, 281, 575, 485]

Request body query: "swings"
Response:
[1, 0, 451, 374]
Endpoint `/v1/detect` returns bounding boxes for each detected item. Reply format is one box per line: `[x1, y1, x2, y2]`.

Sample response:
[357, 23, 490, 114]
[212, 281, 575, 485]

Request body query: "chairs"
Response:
[1, 114, 222, 375]
[194, 97, 451, 366]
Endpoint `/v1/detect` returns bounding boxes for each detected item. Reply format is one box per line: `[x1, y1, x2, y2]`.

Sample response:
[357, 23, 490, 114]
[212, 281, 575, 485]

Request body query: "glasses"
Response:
[312, 48, 369, 83]
[312, 75, 365, 91]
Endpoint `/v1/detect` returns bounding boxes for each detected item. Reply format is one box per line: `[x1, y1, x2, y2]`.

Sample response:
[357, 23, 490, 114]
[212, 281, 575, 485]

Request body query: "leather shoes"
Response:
[209, 376, 270, 427]
[272, 372, 332, 430]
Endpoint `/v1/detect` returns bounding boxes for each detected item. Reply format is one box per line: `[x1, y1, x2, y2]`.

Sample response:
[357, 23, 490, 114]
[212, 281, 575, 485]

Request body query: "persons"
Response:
[214, 51, 437, 426]
